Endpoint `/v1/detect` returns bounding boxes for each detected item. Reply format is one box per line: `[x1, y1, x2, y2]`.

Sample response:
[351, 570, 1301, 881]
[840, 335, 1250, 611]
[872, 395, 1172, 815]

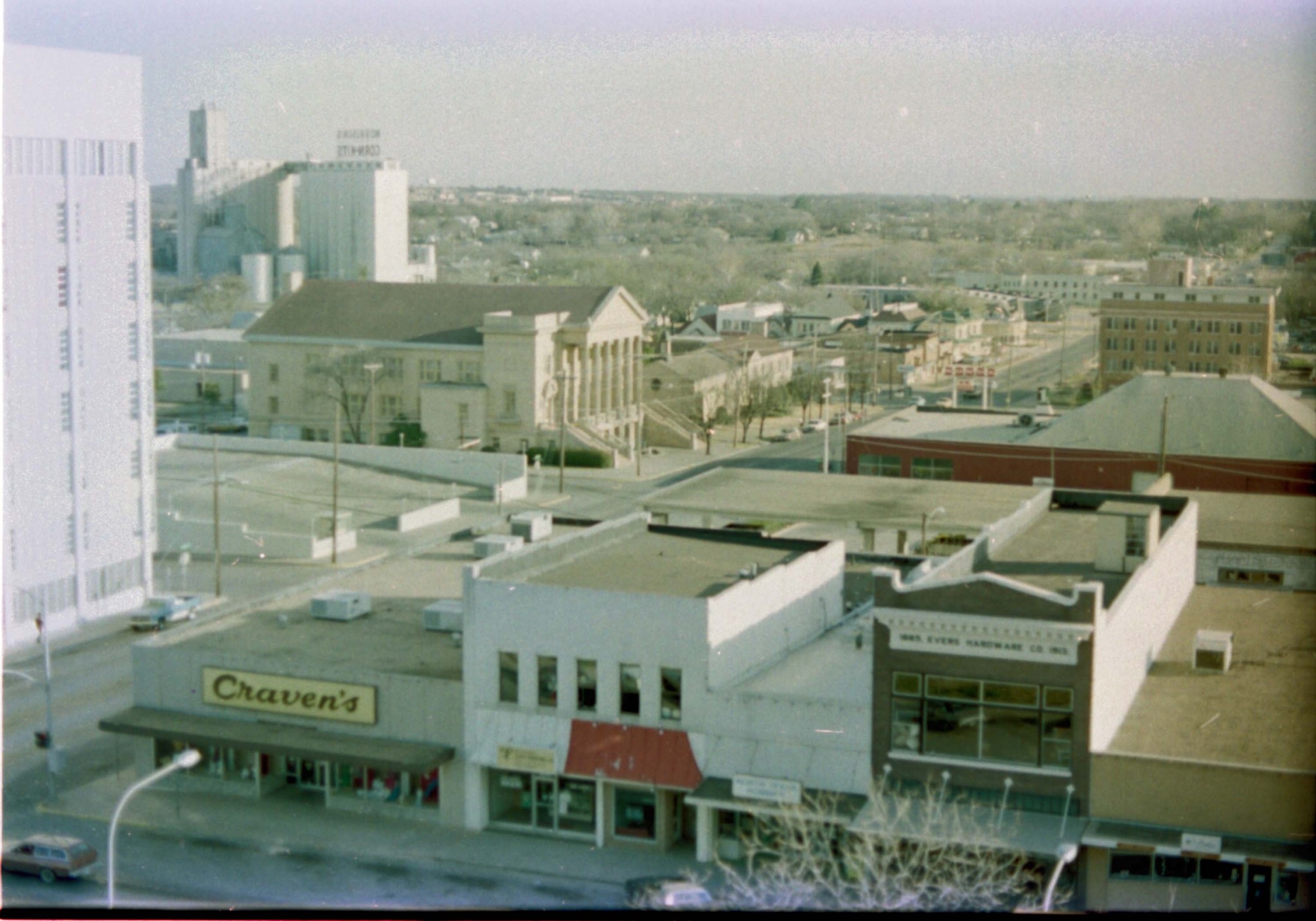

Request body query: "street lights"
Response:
[108, 749, 202, 909]
[1039, 842, 1077, 913]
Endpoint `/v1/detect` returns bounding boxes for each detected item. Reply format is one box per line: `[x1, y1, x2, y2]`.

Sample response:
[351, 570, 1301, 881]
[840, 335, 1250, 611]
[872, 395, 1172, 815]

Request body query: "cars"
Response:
[829, 407, 871, 425]
[207, 417, 248, 433]
[802, 419, 827, 433]
[778, 428, 801, 442]
[1, 835, 100, 885]
[934, 379, 997, 408]
[624, 878, 712, 910]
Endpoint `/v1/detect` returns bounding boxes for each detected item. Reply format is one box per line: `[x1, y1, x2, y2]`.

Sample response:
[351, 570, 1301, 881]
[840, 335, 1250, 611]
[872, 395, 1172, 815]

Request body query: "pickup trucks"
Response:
[128, 593, 203, 631]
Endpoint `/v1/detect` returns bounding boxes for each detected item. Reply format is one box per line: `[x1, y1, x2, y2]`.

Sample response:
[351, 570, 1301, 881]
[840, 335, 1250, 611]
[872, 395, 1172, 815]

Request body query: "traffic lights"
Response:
[34, 730, 49, 748]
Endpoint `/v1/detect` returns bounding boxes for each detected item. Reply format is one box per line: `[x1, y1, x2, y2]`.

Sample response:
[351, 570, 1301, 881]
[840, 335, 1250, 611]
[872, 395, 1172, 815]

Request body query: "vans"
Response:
[157, 422, 198, 435]
